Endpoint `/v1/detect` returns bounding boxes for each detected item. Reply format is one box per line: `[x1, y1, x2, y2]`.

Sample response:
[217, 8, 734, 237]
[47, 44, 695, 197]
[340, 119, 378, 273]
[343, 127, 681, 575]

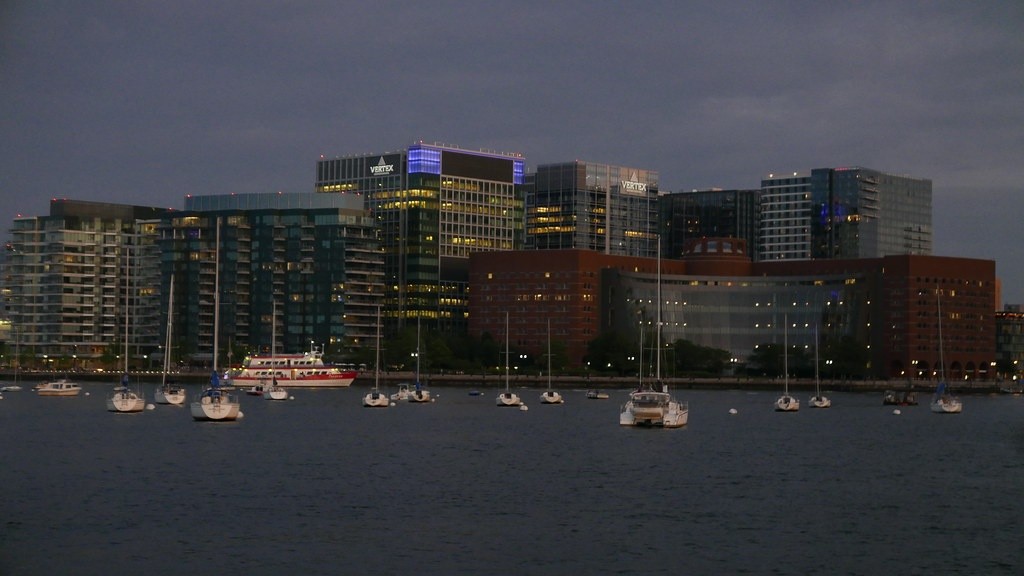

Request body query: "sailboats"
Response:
[809, 323, 831, 408]
[408, 316, 432, 403]
[772, 312, 800, 411]
[539, 318, 562, 404]
[619, 233, 690, 429]
[931, 285, 962, 413]
[0, 329, 23, 393]
[263, 299, 287, 400]
[153, 274, 186, 404]
[190, 217, 242, 423]
[362, 305, 390, 406]
[104, 246, 146, 414]
[495, 311, 521, 406]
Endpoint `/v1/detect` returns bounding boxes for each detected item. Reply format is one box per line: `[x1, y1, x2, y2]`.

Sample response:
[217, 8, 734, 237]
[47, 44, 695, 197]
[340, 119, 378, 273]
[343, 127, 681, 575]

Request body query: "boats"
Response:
[246, 386, 264, 395]
[32, 379, 83, 396]
[231, 352, 358, 387]
[585, 392, 609, 400]
